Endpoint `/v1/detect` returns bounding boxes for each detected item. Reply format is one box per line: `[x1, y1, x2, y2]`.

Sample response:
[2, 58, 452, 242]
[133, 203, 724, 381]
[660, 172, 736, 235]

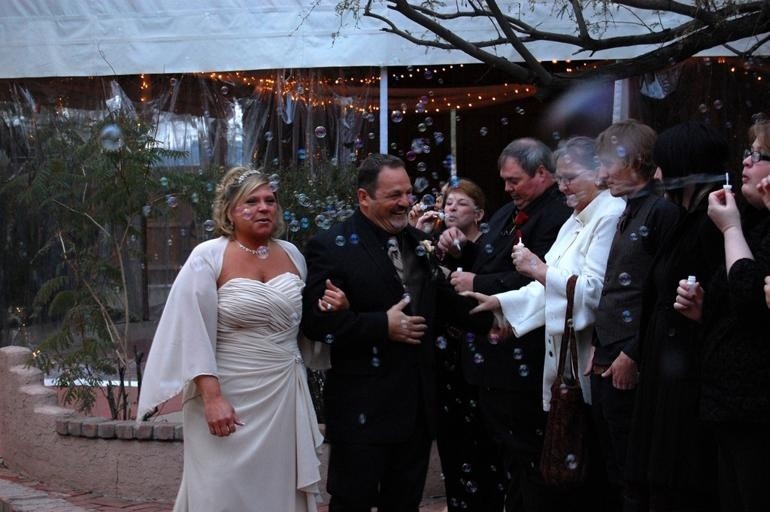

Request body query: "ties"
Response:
[387, 236, 403, 283]
[613, 199, 635, 243]
[504, 208, 520, 237]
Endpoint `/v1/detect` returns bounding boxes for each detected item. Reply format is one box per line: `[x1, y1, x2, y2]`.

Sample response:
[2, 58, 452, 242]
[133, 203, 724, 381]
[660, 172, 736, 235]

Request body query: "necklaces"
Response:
[231, 236, 272, 254]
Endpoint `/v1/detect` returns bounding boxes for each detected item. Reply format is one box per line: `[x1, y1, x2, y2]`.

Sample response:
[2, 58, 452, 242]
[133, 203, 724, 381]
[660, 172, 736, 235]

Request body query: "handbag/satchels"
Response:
[538, 275, 595, 490]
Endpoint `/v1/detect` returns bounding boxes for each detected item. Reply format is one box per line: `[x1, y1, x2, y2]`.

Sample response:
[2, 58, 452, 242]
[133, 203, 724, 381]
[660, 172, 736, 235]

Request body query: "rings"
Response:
[327, 304, 332, 310]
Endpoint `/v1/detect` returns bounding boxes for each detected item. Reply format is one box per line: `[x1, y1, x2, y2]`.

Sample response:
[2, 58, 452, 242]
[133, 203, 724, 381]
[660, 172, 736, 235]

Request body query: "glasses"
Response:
[742, 145, 770, 164]
[556, 170, 588, 186]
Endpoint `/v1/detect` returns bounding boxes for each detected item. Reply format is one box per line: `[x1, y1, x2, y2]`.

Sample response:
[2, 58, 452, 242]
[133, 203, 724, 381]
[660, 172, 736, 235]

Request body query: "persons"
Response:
[752, 169, 770, 303]
[417, 179, 508, 511]
[458, 135, 629, 427]
[439, 139, 573, 512]
[407, 181, 452, 227]
[624, 123, 745, 512]
[302, 153, 510, 511]
[584, 120, 689, 512]
[675, 117, 770, 512]
[131, 167, 349, 512]
